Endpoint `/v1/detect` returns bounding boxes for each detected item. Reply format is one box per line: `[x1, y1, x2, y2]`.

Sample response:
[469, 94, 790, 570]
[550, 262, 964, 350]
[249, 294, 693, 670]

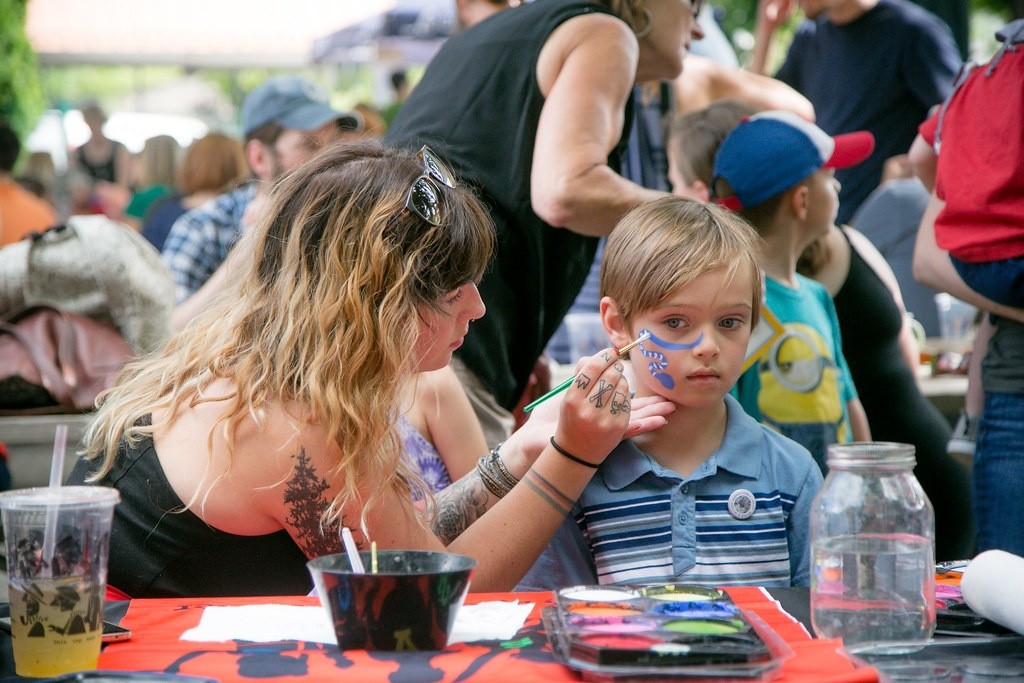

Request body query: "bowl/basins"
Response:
[304, 544, 478, 654]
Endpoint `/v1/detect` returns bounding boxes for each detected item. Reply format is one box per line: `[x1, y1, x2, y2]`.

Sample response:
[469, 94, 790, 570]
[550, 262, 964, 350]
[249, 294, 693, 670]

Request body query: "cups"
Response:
[1, 486, 122, 679]
[932, 289, 978, 347]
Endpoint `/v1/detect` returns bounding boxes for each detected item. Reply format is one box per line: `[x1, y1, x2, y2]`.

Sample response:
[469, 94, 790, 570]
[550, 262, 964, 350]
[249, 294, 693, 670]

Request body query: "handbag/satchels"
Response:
[0, 302, 135, 415]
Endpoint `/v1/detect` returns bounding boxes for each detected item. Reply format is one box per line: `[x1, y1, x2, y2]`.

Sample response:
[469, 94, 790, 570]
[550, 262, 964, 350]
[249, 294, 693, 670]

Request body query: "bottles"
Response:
[807, 440, 936, 657]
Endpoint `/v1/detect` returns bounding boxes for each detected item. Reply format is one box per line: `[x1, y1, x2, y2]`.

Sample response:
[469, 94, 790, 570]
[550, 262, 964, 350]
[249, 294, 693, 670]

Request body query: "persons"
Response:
[0, 0, 1024, 600]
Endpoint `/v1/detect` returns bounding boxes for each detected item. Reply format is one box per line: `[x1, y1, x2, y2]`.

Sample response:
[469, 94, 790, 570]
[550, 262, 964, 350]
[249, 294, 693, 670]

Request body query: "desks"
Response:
[0, 588, 1024, 683]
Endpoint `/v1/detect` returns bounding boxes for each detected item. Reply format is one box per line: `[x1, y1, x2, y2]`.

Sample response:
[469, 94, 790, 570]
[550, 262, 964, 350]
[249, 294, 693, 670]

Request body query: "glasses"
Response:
[379, 144, 456, 296]
[693, 0, 702, 18]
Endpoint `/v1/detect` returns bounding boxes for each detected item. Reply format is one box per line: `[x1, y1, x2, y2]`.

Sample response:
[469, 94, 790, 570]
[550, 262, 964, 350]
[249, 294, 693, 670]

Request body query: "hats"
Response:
[711, 111, 876, 212]
[240, 76, 359, 134]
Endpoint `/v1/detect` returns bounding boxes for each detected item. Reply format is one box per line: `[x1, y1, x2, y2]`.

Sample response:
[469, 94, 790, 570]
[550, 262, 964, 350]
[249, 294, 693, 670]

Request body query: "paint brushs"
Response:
[371, 541, 378, 574]
[342, 527, 365, 573]
[523, 332, 652, 413]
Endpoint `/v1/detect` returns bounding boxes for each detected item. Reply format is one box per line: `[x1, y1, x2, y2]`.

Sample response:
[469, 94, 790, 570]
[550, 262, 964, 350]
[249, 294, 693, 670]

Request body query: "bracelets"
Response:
[550, 436, 601, 470]
[477, 443, 518, 499]
